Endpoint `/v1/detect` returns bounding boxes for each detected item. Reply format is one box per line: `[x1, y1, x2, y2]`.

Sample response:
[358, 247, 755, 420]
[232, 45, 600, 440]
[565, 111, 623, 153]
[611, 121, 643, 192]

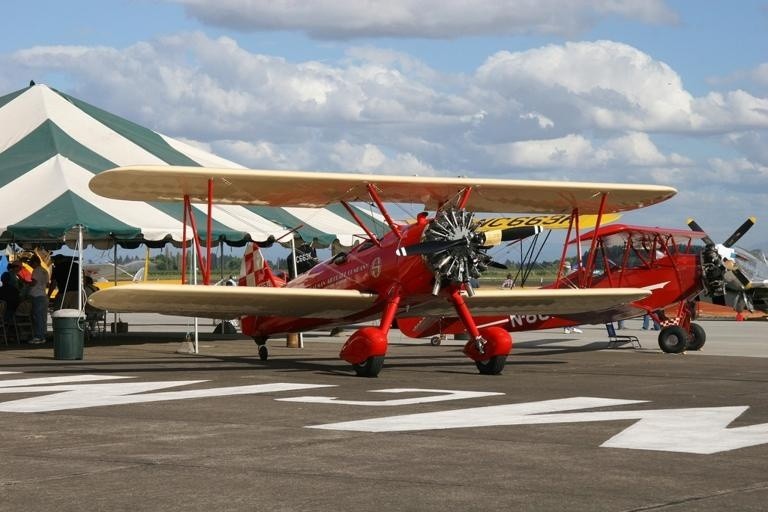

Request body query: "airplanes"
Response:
[396, 216, 759, 352]
[87, 168, 677, 374]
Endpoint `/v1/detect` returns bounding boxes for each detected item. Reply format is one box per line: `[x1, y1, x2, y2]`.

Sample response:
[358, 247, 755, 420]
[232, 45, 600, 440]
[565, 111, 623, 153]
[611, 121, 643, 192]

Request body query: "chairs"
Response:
[0, 298, 106, 346]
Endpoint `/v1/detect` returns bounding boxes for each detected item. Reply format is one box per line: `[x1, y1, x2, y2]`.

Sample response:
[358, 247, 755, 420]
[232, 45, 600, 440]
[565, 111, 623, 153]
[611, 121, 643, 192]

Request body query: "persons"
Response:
[46, 253, 97, 344]
[7, 259, 24, 341]
[617, 320, 629, 330]
[84, 275, 106, 333]
[227, 275, 237, 287]
[639, 314, 661, 331]
[502, 272, 517, 288]
[22, 254, 50, 345]
[0, 272, 21, 344]
[561, 260, 583, 334]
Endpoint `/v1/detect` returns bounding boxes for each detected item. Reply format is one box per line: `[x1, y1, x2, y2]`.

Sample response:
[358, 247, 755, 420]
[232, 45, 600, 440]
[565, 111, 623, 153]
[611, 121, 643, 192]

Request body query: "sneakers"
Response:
[28, 337, 47, 344]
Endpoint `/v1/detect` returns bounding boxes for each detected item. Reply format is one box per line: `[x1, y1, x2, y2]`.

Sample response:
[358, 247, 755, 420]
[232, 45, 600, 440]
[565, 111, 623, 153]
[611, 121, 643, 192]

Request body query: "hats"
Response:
[26, 255, 41, 264]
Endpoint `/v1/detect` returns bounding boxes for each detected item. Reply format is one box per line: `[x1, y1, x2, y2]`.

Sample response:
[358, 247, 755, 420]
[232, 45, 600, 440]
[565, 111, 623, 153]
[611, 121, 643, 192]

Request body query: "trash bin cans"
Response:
[51, 309, 87, 360]
